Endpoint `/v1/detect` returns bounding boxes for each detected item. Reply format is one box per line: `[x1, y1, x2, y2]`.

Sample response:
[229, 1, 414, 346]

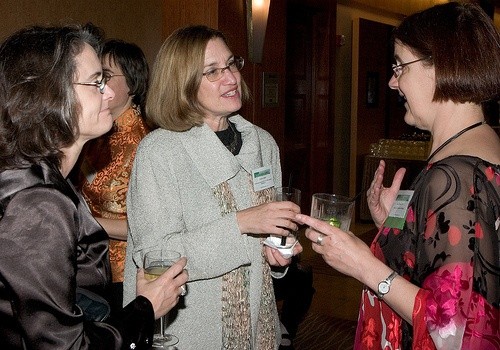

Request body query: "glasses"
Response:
[392, 56, 426, 78]
[103, 72, 124, 81]
[73, 76, 107, 94]
[202, 55, 244, 82]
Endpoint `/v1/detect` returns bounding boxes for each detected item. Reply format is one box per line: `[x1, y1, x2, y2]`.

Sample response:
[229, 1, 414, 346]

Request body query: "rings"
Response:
[317, 234, 325, 244]
[181, 285, 187, 296]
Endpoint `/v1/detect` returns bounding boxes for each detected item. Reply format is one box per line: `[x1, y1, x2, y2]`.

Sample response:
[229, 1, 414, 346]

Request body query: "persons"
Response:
[293, 0, 500, 350]
[0, 21, 189, 350]
[120, 25, 300, 350]
[80, 38, 149, 283]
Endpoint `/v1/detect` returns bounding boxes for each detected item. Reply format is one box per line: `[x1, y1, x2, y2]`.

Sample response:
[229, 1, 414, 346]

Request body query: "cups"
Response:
[309, 192, 356, 234]
[271, 185, 301, 249]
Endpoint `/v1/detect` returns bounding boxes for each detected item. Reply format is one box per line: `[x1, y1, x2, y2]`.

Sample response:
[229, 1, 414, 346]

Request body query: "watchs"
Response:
[377, 272, 399, 298]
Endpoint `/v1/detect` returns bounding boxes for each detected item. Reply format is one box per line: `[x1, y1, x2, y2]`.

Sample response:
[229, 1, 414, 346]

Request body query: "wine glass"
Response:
[143, 249, 181, 348]
